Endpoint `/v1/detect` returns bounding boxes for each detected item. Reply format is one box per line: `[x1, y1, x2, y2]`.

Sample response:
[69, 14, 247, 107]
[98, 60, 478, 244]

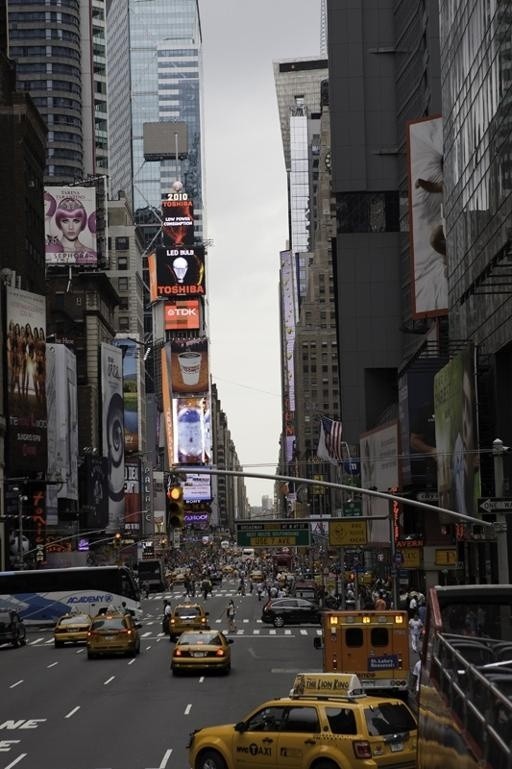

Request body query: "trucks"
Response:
[314, 606, 411, 703]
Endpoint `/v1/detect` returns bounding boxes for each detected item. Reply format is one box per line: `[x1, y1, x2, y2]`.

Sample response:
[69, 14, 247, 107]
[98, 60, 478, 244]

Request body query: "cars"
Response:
[173, 541, 297, 588]
[171, 630, 235, 677]
[53, 603, 93, 646]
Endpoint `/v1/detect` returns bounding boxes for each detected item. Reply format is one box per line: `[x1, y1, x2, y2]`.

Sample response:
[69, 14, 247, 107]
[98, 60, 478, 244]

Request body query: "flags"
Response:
[315, 414, 344, 466]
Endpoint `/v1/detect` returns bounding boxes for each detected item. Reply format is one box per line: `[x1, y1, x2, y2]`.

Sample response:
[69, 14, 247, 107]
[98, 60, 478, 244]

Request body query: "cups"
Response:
[177, 351, 204, 386]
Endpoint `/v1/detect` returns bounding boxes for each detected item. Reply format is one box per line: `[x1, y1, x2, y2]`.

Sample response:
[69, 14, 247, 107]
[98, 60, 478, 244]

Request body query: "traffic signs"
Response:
[478, 497, 512, 513]
[236, 524, 310, 547]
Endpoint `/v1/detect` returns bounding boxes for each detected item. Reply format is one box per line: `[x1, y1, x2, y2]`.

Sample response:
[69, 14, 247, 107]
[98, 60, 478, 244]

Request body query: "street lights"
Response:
[13, 487, 28, 563]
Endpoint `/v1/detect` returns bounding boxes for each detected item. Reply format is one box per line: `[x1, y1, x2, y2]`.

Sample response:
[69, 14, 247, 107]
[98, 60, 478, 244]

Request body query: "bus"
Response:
[418, 585, 512, 768]
[0, 565, 143, 627]
[138, 558, 165, 592]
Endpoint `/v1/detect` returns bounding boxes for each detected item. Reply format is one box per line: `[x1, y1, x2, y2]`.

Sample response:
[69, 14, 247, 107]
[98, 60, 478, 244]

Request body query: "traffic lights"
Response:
[168, 482, 184, 529]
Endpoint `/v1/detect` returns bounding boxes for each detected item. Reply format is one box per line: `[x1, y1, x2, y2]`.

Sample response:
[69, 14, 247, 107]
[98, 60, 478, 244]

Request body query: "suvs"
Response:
[0, 606, 26, 647]
[262, 598, 334, 630]
[167, 602, 209, 638]
[85, 603, 143, 660]
[186, 671, 419, 767]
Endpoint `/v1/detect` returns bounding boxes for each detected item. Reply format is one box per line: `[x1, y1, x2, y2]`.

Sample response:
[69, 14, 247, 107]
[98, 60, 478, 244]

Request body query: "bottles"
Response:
[179, 399, 211, 465]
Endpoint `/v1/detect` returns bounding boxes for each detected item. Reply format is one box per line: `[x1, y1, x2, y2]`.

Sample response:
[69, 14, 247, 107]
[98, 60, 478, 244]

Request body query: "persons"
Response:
[115, 545, 490, 704]
[436, 350, 480, 524]
[44, 197, 99, 263]
[5, 320, 46, 404]
